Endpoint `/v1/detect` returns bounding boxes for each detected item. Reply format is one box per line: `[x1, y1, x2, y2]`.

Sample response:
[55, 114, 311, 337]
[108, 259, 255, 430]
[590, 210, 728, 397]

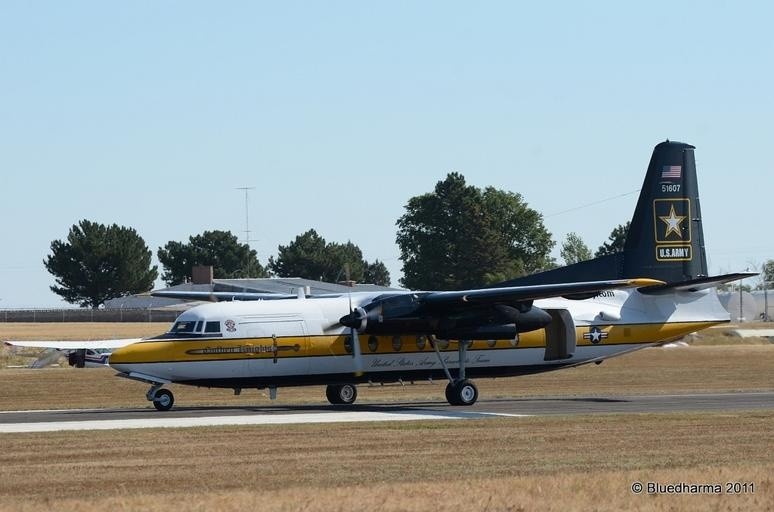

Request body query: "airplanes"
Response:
[3, 338, 141, 369]
[110, 139, 764, 411]
